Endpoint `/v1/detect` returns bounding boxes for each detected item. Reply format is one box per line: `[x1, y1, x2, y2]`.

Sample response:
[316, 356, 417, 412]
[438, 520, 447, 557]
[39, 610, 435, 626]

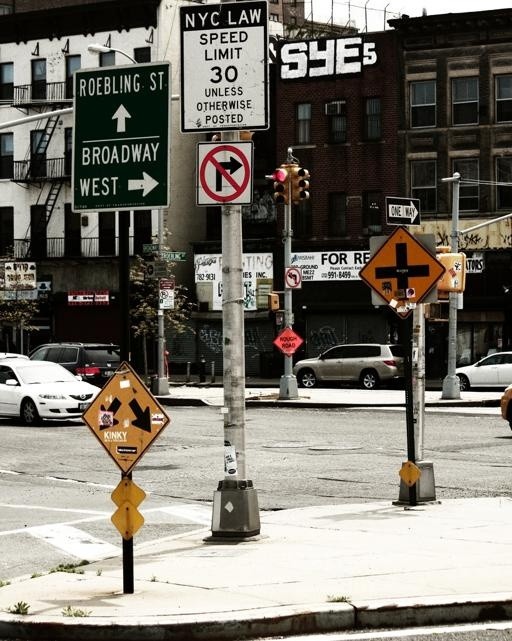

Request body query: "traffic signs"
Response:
[143, 243, 160, 251]
[160, 251, 189, 261]
[70, 61, 171, 215]
[145, 260, 169, 278]
[179, 1, 271, 134]
[384, 196, 422, 228]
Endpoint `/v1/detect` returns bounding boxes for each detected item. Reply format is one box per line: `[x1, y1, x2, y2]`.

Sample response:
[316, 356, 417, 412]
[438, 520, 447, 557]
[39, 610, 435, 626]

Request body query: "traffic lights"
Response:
[273, 168, 290, 206]
[292, 168, 310, 202]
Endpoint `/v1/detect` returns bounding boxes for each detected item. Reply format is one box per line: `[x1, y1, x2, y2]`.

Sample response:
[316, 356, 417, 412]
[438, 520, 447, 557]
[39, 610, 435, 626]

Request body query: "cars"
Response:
[0, 359, 102, 426]
[28, 343, 122, 386]
[455, 352, 512, 391]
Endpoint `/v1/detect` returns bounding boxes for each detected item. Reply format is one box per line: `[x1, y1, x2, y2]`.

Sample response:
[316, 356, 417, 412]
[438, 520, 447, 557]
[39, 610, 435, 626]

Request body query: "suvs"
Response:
[293, 343, 404, 391]
